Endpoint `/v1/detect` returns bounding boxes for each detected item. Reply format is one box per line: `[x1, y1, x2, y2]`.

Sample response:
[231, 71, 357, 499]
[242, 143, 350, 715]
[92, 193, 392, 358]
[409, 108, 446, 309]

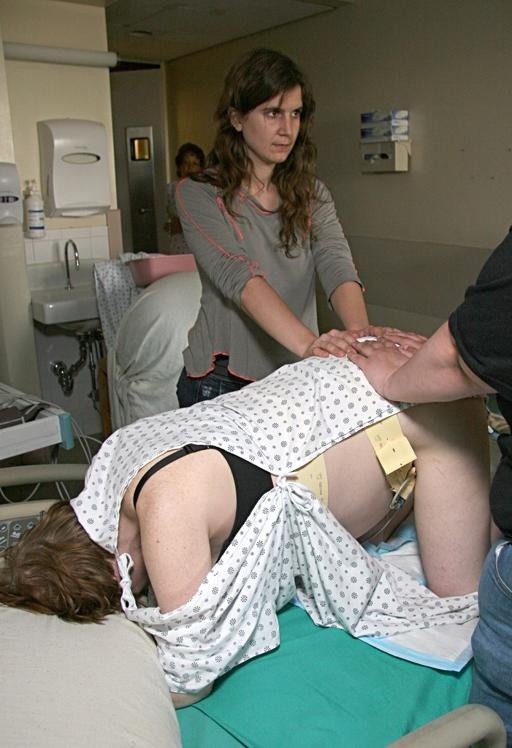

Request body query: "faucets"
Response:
[64, 239, 80, 291]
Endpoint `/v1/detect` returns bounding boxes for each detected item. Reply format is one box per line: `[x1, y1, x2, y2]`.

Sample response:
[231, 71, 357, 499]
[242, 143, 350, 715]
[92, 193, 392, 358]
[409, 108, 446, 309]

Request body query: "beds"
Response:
[0, 464, 511, 748]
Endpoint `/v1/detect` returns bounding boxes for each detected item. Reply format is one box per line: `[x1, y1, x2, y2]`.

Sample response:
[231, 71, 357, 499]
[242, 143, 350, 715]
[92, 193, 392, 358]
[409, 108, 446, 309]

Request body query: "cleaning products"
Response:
[21, 179, 45, 238]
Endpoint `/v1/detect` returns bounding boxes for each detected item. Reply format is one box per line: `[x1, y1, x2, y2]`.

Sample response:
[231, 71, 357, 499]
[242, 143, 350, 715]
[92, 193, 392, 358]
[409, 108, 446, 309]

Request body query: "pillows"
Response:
[0, 603, 183, 748]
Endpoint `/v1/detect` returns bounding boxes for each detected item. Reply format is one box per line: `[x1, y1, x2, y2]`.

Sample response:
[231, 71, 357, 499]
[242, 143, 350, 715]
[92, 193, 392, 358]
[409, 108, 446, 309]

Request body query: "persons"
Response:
[354, 227, 511, 740]
[162, 139, 208, 254]
[171, 46, 422, 409]
[0, 326, 503, 710]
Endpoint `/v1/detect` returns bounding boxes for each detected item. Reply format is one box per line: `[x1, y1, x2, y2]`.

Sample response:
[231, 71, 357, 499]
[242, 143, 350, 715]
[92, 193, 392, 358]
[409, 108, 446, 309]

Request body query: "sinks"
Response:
[32, 288, 101, 335]
